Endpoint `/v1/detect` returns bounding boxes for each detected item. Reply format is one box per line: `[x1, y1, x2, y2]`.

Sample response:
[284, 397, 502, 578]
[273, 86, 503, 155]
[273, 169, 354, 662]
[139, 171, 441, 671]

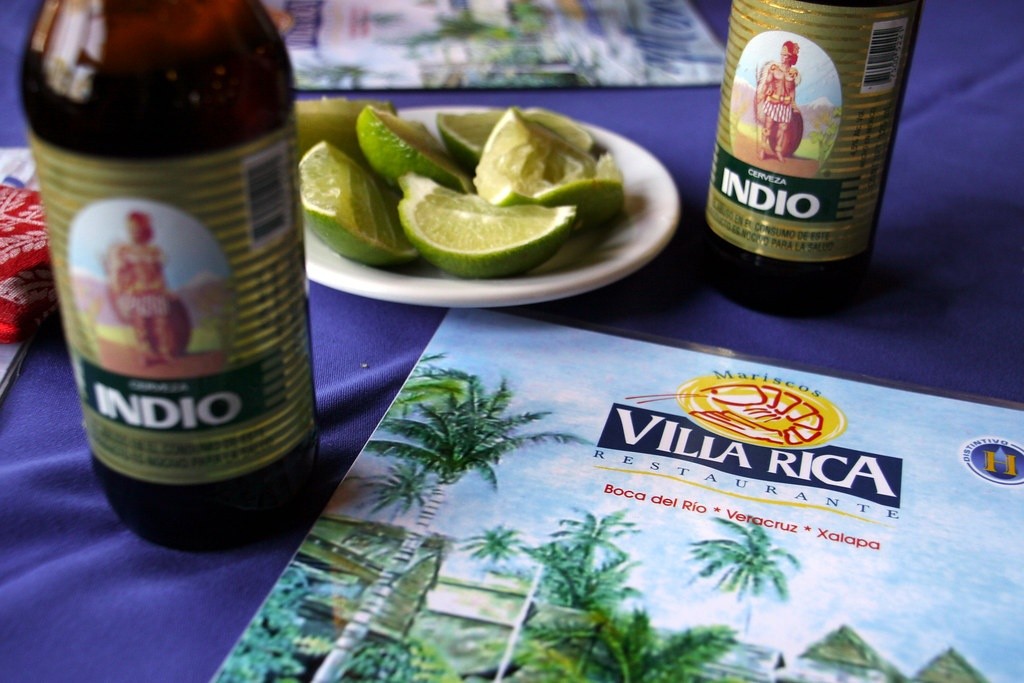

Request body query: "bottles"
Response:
[698, 0, 921, 318]
[24, 0, 319, 554]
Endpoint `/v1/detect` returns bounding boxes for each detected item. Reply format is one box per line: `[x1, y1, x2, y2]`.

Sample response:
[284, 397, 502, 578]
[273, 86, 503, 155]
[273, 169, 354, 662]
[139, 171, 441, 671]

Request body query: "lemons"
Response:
[299, 105, 624, 275]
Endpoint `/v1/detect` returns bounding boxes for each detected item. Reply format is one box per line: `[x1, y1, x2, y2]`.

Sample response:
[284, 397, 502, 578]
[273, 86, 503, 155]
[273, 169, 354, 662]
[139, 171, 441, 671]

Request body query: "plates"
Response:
[306, 104, 683, 308]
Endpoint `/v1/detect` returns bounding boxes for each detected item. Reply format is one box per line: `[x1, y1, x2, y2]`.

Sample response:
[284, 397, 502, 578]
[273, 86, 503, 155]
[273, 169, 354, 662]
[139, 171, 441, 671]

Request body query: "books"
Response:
[208, 309, 1023, 682]
[263, 0, 733, 95]
